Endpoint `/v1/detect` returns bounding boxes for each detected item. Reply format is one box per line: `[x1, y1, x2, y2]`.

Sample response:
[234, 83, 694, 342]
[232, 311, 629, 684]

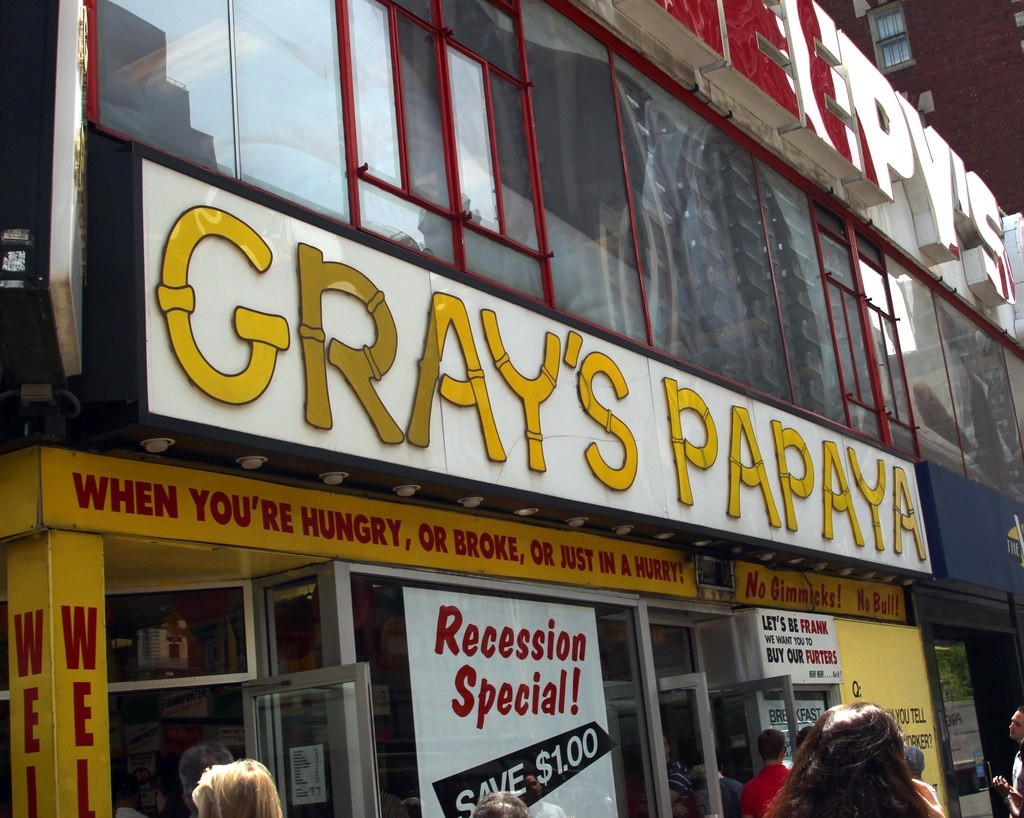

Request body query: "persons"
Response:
[111, 741, 283, 818]
[992, 707, 1024, 818]
[662, 701, 947, 818]
[380, 773, 613, 818]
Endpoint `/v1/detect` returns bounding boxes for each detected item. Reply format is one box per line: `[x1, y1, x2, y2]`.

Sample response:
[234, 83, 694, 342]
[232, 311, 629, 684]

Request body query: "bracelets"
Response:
[1004, 797, 1012, 803]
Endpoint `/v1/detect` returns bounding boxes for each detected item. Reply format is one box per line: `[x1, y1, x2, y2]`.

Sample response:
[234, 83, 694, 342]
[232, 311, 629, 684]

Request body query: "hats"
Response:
[903, 747, 925, 773]
